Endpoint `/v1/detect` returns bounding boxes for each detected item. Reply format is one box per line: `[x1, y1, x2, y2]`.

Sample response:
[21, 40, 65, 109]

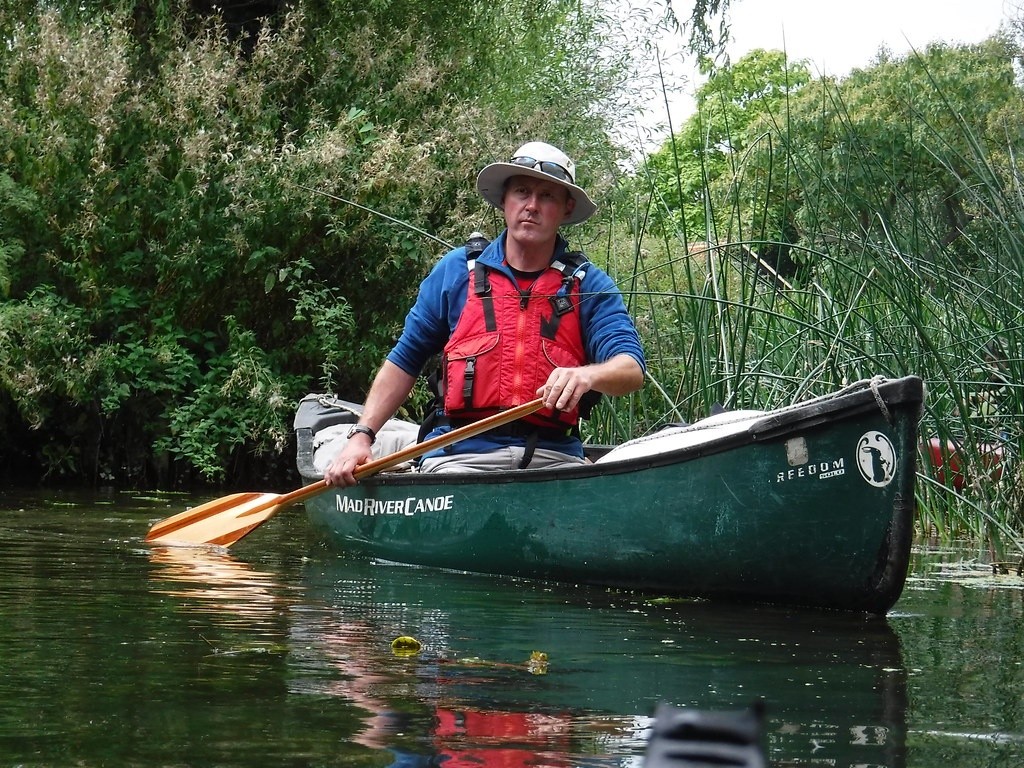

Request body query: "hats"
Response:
[477, 142, 597, 226]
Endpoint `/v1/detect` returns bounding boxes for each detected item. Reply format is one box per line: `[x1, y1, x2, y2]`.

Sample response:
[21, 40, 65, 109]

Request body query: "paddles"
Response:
[145, 394, 543, 550]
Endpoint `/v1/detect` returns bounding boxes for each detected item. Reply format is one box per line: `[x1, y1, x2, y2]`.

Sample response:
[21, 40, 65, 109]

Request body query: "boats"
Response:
[917, 434, 1011, 491]
[292, 373, 924, 615]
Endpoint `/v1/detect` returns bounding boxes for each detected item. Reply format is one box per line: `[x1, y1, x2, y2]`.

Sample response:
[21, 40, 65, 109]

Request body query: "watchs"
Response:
[347, 423, 376, 447]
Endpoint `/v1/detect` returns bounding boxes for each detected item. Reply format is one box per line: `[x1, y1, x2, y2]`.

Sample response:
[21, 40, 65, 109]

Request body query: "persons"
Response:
[323, 142, 646, 490]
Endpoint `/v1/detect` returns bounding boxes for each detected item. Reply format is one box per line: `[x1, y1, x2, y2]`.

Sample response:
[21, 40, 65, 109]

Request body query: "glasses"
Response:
[510, 157, 574, 184]
